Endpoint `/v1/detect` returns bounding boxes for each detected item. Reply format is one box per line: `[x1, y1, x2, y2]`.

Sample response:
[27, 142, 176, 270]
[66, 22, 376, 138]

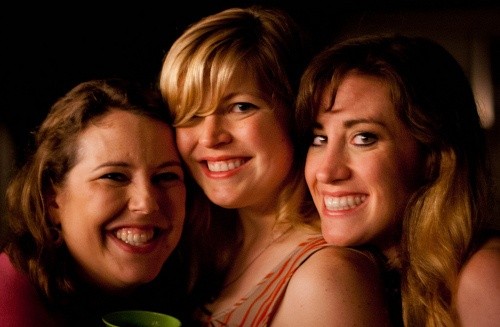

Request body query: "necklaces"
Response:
[221, 224, 293, 290]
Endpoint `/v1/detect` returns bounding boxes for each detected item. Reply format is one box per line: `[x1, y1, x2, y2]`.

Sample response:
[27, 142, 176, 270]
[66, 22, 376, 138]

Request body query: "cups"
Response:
[101, 310, 181, 327]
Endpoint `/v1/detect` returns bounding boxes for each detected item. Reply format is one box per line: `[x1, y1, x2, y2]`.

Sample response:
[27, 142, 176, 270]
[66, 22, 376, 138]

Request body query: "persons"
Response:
[162, 8, 394, 327]
[0, 73, 211, 327]
[292, 35, 500, 327]
